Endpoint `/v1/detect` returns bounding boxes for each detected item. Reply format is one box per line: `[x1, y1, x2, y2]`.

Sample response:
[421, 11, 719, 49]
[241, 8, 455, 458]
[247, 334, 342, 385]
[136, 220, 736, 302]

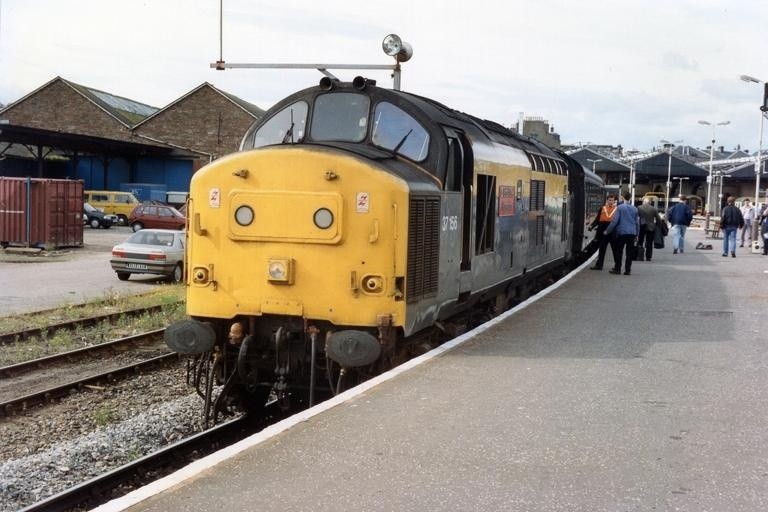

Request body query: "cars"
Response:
[109, 228, 185, 282]
[84, 202, 119, 230]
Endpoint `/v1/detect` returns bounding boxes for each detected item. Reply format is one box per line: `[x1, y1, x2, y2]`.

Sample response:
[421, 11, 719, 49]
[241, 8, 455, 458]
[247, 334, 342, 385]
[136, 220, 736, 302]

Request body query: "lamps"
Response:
[382, 33, 411, 67]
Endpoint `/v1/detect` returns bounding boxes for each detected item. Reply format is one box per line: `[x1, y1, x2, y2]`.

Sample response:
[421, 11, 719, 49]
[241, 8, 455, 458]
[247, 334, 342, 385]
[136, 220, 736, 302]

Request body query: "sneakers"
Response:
[609, 267, 621, 274]
[624, 271, 631, 275]
[590, 266, 603, 270]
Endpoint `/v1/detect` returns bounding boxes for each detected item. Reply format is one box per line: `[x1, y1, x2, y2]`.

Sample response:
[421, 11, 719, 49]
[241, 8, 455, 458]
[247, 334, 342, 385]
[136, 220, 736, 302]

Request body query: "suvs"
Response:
[129, 203, 185, 232]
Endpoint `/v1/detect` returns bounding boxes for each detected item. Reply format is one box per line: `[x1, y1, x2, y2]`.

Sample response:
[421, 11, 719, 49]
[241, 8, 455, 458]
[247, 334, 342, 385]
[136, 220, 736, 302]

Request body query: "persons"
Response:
[739, 198, 768, 255]
[720, 197, 744, 257]
[636, 196, 663, 261]
[590, 195, 621, 270]
[603, 190, 640, 276]
[666, 194, 693, 254]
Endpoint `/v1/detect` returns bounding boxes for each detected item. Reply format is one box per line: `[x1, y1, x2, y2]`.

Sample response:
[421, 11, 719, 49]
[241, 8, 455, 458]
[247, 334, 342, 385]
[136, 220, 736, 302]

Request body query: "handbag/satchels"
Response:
[631, 240, 645, 261]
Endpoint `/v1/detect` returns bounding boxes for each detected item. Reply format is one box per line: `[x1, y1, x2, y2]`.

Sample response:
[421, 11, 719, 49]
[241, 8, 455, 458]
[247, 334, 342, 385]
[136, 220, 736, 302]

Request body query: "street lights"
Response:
[658, 138, 685, 211]
[622, 150, 641, 202]
[585, 157, 603, 173]
[738, 74, 765, 252]
[672, 175, 690, 197]
[712, 172, 731, 213]
[695, 119, 733, 236]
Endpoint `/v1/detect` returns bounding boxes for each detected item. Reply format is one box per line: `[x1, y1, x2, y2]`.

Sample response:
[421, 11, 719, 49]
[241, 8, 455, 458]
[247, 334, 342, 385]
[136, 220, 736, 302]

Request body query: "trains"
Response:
[167, 35, 607, 429]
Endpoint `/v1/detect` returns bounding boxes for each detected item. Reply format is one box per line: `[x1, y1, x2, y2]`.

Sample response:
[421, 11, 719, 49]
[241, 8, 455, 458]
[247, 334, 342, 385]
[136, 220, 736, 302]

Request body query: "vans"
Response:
[84, 189, 140, 227]
